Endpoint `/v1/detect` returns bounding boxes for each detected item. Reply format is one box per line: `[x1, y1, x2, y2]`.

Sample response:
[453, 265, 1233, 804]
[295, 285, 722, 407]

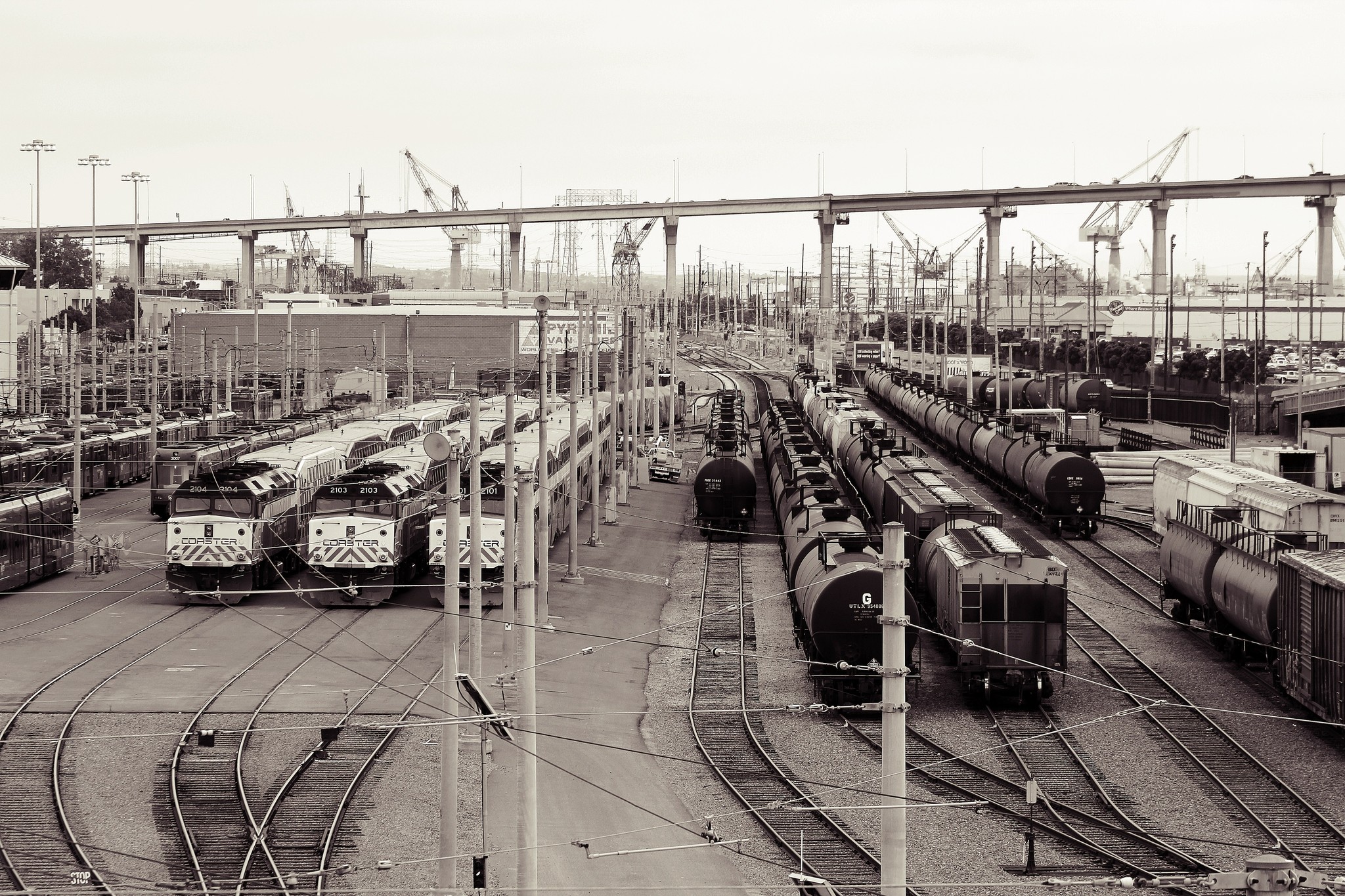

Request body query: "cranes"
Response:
[401, 148, 483, 292]
[1073, 125, 1198, 299]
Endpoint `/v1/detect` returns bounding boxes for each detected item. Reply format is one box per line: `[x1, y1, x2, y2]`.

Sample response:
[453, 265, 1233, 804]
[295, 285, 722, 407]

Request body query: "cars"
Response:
[1092, 333, 1345, 386]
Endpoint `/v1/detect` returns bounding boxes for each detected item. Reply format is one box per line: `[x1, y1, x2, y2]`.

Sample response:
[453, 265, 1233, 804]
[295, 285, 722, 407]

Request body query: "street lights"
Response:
[20, 139, 151, 524]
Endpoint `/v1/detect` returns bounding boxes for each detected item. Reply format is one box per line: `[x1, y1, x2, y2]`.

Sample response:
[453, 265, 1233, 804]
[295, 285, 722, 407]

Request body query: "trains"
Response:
[1151, 449, 1345, 733]
[784, 359, 1069, 719]
[147, 385, 372, 521]
[758, 396, 921, 712]
[694, 386, 757, 537]
[865, 350, 1106, 541]
[944, 369, 1112, 429]
[163, 392, 618, 608]
[0, 403, 250, 598]
